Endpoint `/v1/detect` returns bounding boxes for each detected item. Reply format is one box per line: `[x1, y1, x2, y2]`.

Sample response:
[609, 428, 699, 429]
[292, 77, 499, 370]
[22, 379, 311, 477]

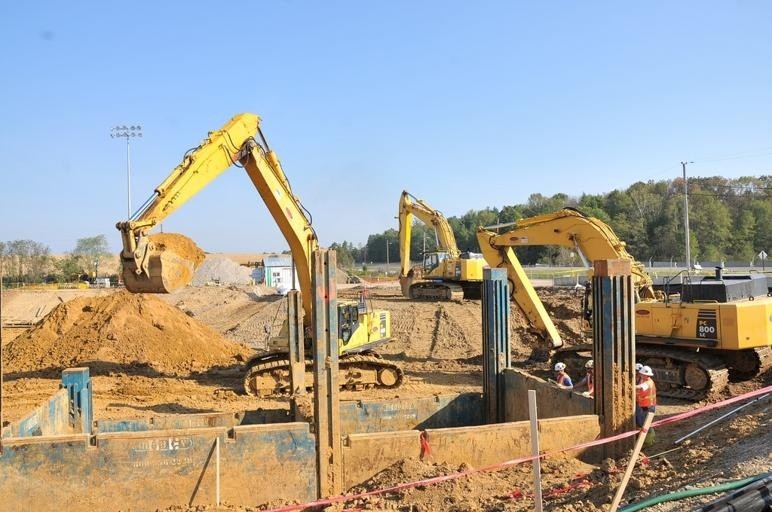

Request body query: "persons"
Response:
[636, 362, 644, 385]
[559, 359, 594, 396]
[554, 362, 574, 390]
[636, 365, 657, 433]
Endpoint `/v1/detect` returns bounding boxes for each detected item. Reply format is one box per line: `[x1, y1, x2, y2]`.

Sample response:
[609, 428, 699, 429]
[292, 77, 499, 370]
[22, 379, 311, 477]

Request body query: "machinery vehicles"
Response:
[116, 112, 404, 397]
[397, 191, 492, 303]
[476, 206, 771, 402]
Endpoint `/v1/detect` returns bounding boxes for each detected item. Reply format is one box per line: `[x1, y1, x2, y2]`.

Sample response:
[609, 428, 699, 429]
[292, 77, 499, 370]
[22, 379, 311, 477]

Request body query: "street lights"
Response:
[109, 124, 143, 219]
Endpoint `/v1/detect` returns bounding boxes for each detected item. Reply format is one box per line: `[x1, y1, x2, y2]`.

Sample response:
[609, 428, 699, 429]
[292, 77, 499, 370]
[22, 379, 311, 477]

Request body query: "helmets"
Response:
[636, 363, 644, 371]
[585, 359, 594, 369]
[555, 362, 567, 371]
[639, 365, 654, 376]
